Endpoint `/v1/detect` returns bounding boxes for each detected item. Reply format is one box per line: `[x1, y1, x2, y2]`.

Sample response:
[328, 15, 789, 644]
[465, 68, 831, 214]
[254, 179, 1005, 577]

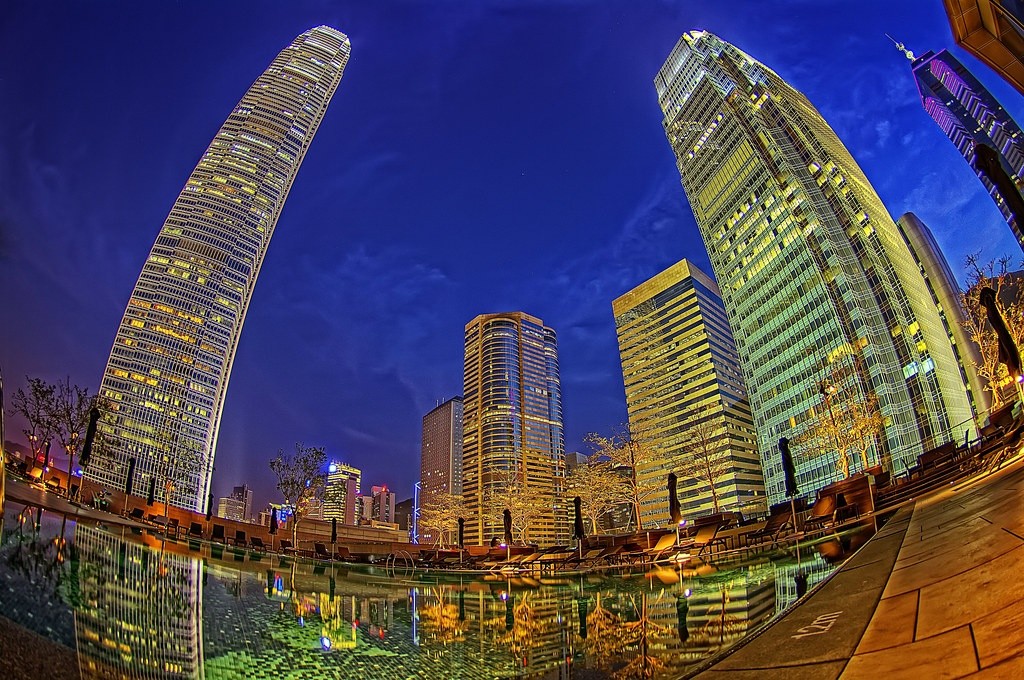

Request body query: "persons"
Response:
[96, 487, 114, 515]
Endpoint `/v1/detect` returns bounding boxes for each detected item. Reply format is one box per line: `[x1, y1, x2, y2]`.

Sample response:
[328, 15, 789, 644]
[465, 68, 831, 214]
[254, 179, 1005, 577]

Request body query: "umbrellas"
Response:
[572, 496, 585, 558]
[266, 507, 279, 550]
[503, 508, 516, 564]
[40, 441, 55, 483]
[778, 437, 800, 533]
[70, 406, 101, 500]
[117, 458, 136, 515]
[458, 516, 465, 564]
[143, 476, 157, 520]
[978, 286, 1023, 405]
[665, 473, 685, 547]
[328, 516, 338, 558]
[202, 493, 215, 539]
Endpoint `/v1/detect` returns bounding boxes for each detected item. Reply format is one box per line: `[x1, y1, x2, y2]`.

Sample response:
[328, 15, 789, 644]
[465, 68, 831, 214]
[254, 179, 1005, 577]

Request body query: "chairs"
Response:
[3, 402, 1024, 576]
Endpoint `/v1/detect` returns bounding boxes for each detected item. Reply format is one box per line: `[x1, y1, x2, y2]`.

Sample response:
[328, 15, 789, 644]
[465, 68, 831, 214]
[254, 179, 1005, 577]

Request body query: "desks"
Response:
[709, 535, 732, 553]
[177, 525, 189, 534]
[298, 548, 317, 559]
[737, 530, 763, 545]
[531, 557, 565, 571]
[837, 502, 859, 525]
[227, 537, 236, 545]
[621, 551, 644, 564]
